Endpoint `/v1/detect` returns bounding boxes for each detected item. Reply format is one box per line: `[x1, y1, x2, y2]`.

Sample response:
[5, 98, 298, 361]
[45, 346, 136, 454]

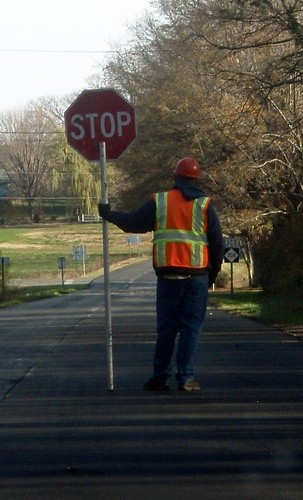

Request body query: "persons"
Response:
[97, 157, 225, 392]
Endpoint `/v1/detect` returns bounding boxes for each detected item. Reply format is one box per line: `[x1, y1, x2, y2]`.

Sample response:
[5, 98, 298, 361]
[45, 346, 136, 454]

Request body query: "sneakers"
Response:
[145, 379, 171, 391]
[178, 382, 201, 391]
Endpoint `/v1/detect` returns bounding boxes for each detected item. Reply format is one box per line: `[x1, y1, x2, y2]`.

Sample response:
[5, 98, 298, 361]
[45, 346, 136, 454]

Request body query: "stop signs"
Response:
[62, 89, 138, 164]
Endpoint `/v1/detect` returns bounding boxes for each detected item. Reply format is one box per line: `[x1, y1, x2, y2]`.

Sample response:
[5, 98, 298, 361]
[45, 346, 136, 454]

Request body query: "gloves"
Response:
[209, 277, 215, 289]
[98, 202, 112, 218]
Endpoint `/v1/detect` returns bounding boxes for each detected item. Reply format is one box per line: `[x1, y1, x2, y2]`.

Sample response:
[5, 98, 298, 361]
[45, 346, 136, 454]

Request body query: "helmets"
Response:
[175, 157, 201, 179]
